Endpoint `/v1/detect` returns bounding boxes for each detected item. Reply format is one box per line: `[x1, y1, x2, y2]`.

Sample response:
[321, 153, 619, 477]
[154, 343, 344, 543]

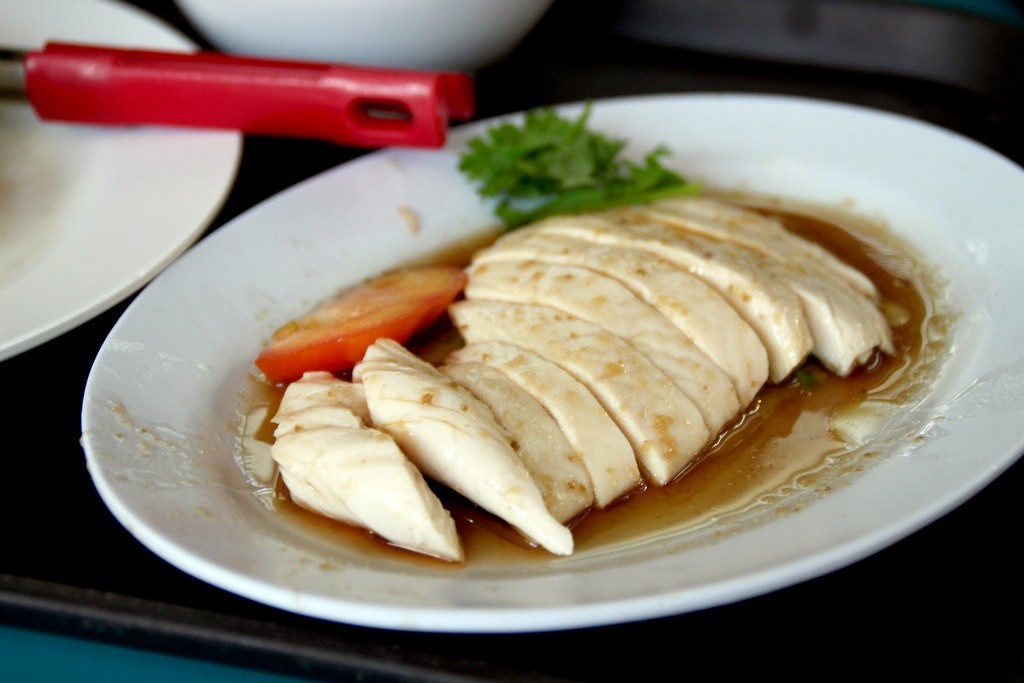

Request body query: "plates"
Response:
[81, 93, 1024, 630]
[0, 0, 243, 359]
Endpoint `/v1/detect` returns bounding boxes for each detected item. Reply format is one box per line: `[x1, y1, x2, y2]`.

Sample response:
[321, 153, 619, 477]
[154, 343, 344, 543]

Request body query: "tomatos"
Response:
[256, 264, 468, 380]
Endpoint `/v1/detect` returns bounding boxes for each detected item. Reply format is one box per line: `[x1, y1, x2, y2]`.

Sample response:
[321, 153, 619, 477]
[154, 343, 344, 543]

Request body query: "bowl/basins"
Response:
[175, 1, 551, 72]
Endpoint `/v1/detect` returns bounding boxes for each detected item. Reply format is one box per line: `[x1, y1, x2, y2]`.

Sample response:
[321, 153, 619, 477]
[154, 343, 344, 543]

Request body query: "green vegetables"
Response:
[459, 99, 697, 228]
